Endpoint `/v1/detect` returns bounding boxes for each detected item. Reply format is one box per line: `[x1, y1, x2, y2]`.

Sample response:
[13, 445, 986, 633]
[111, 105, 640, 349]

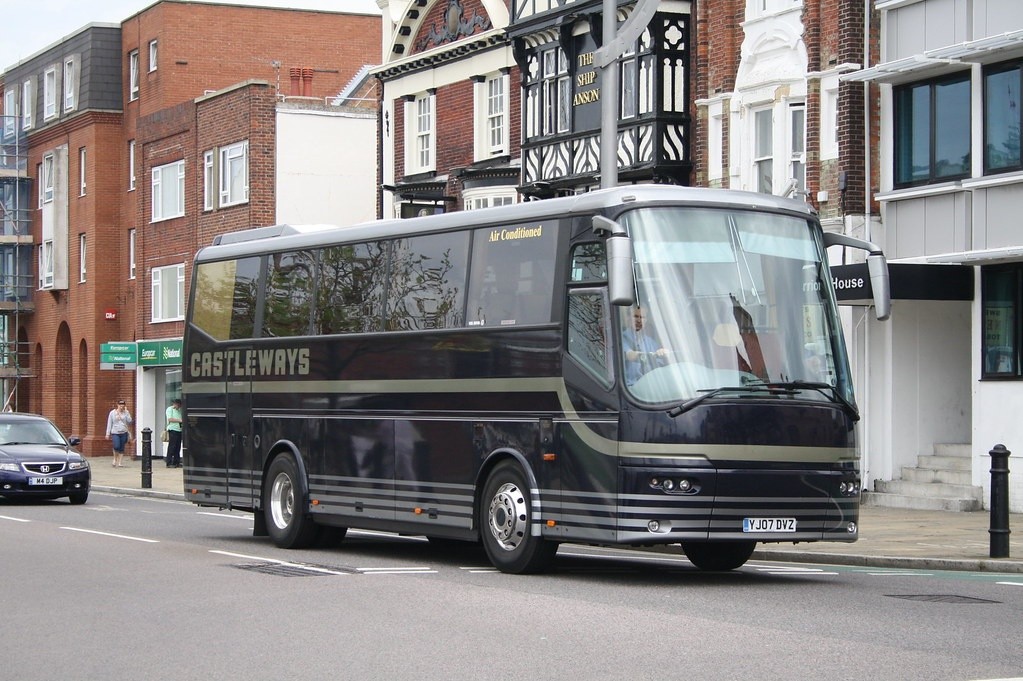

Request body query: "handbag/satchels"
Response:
[161, 430, 169, 442]
[127, 431, 132, 445]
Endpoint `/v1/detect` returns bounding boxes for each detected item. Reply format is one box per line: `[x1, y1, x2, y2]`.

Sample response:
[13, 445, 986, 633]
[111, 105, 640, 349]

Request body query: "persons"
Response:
[621, 301, 670, 384]
[105, 400, 132, 467]
[166, 399, 183, 468]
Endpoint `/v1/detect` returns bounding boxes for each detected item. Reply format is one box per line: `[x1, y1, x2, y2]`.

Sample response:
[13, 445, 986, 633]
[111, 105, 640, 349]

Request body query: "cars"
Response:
[0, 412, 92, 506]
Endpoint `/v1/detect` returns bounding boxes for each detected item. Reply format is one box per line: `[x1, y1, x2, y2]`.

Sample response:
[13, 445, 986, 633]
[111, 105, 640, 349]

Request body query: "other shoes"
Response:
[112, 460, 115, 467]
[118, 462, 122, 466]
[166, 461, 183, 469]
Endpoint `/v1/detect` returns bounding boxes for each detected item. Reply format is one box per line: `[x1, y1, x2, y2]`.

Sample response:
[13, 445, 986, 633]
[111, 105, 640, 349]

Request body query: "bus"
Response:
[181, 184, 891, 575]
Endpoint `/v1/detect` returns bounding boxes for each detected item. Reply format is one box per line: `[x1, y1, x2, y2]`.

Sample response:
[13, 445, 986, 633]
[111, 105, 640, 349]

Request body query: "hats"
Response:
[118, 400, 125, 405]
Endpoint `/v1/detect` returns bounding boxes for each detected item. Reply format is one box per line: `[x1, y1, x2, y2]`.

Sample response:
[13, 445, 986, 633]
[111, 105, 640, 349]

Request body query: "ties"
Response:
[637, 334, 650, 377]
